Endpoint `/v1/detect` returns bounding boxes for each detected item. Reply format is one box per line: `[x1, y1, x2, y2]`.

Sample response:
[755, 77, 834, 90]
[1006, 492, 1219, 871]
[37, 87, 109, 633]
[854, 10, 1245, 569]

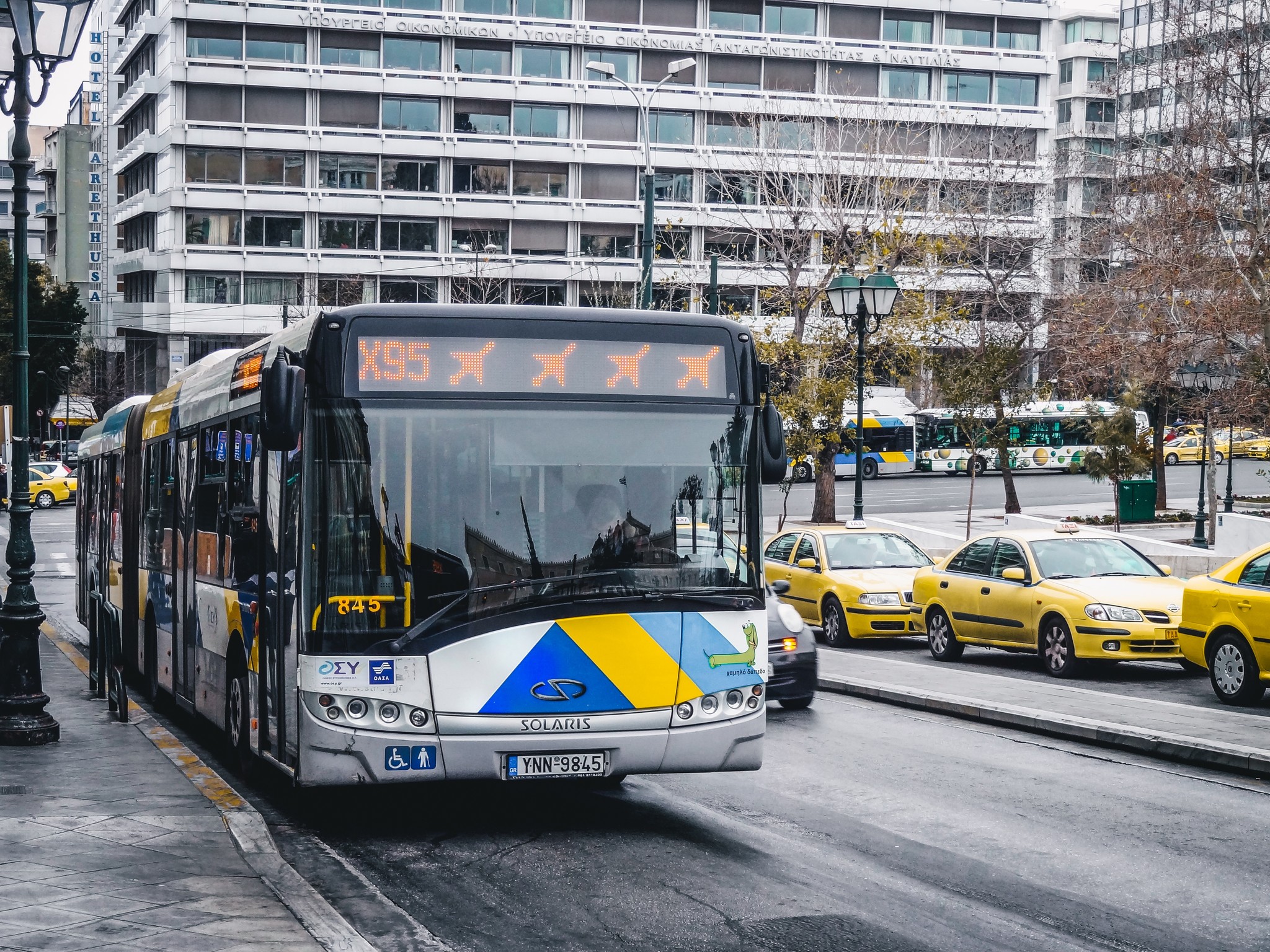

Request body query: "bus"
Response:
[782, 411, 918, 484]
[71, 299, 789, 809]
[914, 399, 1153, 478]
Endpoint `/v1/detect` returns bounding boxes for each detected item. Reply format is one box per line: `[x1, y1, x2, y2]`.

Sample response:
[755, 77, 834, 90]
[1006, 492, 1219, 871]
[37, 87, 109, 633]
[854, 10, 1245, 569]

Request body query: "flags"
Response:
[619, 476, 626, 485]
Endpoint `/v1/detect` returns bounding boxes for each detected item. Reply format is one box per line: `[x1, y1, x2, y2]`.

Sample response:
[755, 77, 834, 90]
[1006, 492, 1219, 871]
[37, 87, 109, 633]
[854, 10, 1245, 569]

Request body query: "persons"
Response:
[28, 435, 35, 455]
[0, 465, 7, 513]
[1166, 428, 1178, 442]
[576, 490, 660, 560]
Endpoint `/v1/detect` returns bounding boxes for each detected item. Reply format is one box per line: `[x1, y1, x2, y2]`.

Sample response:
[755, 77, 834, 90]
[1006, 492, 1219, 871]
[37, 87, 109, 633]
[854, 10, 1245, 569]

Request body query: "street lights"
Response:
[583, 57, 699, 313]
[824, 263, 902, 523]
[38, 370, 49, 441]
[58, 366, 70, 467]
[0, 0, 97, 748]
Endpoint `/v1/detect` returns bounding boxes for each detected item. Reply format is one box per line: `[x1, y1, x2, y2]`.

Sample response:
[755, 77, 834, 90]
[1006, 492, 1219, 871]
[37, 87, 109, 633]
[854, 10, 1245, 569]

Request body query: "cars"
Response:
[908, 521, 1192, 679]
[29, 467, 71, 509]
[29, 438, 80, 503]
[1175, 541, 1270, 709]
[1146, 421, 1270, 466]
[674, 515, 819, 710]
[739, 520, 949, 649]
